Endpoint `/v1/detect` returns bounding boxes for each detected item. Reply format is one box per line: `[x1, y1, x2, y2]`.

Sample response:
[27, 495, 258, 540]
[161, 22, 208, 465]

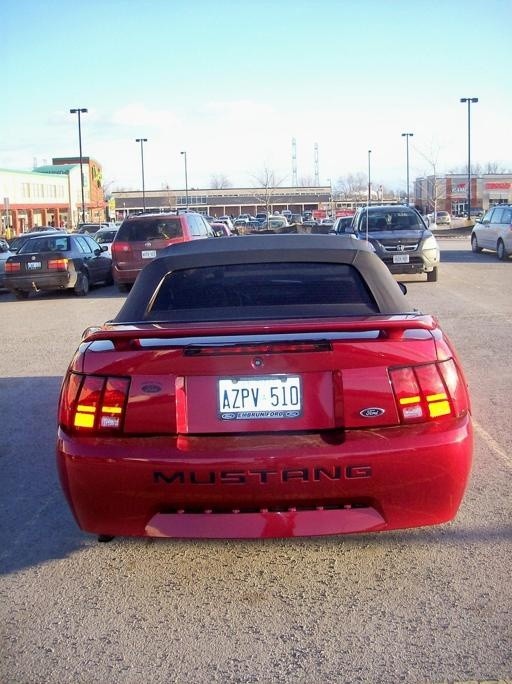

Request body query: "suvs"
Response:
[461, 207, 485, 217]
[344, 205, 440, 281]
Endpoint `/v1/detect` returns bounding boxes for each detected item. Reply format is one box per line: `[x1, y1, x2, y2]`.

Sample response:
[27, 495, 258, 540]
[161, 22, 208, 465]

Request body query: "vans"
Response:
[111, 209, 216, 292]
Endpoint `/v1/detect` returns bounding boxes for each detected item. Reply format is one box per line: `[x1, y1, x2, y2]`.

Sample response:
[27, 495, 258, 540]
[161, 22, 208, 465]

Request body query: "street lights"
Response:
[136, 138, 148, 209]
[70, 108, 88, 223]
[181, 151, 189, 209]
[460, 98, 479, 219]
[369, 150, 371, 205]
[402, 133, 413, 206]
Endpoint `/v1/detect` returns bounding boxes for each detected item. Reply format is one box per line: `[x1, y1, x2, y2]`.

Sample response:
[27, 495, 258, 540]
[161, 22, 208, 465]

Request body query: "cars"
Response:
[4, 233, 112, 300]
[0, 237, 16, 293]
[470, 203, 512, 260]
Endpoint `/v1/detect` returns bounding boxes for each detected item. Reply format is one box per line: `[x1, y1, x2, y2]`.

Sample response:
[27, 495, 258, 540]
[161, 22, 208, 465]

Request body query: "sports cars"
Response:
[56, 232, 471, 543]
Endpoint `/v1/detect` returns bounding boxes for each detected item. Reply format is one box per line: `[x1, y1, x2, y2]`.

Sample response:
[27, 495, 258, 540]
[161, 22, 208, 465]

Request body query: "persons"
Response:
[48, 218, 66, 227]
